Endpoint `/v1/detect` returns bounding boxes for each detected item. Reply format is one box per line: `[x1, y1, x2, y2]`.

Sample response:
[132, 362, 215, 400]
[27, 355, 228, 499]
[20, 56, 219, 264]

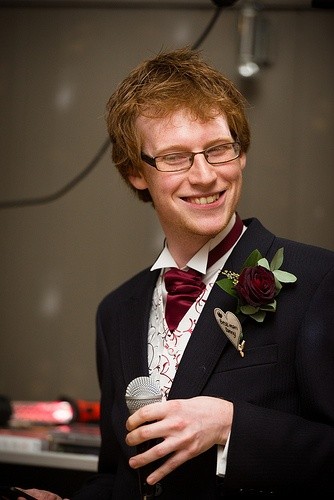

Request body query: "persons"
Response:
[10, 47, 334, 500]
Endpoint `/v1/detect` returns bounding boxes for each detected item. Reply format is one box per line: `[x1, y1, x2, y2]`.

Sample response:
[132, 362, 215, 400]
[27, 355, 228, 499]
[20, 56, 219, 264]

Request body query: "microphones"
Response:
[125, 377, 163, 500]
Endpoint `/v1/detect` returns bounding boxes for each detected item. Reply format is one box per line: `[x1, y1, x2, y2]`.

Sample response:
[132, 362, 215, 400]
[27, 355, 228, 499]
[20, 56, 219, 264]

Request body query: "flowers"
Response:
[220, 247, 298, 325]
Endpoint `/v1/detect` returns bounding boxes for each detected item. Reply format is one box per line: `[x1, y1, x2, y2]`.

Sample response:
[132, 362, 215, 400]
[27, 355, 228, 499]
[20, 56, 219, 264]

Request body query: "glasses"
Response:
[140, 132, 242, 171]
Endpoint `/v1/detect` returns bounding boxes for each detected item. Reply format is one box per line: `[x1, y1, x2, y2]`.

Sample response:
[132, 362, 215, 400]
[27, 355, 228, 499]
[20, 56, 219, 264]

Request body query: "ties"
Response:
[162, 210, 244, 335]
